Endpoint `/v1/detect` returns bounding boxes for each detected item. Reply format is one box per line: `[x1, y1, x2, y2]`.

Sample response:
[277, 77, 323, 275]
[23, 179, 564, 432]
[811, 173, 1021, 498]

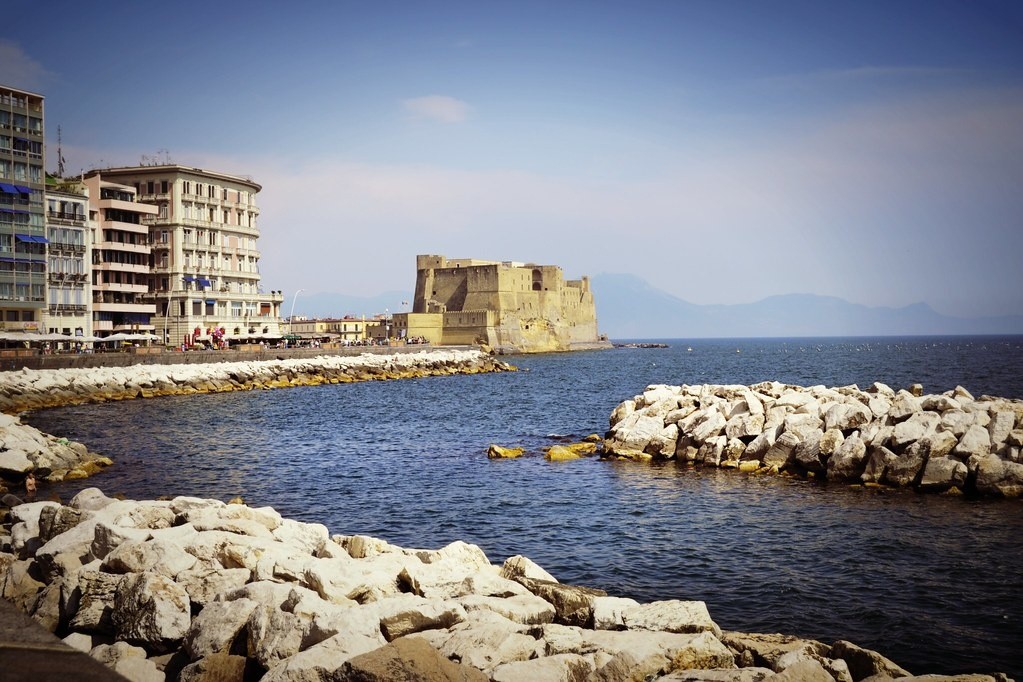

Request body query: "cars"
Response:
[372, 336, 388, 345]
[413, 338, 429, 344]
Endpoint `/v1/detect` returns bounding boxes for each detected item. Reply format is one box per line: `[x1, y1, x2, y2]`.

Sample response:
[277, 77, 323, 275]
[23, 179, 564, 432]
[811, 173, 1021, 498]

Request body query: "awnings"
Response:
[14, 235, 50, 248]
[182, 276, 215, 305]
[14, 136, 33, 143]
[0, 208, 33, 217]
[0, 257, 48, 287]
[0, 183, 33, 195]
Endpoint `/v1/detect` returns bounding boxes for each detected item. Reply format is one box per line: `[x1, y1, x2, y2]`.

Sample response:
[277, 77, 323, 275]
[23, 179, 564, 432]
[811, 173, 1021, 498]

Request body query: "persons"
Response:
[181, 336, 427, 352]
[26, 342, 133, 354]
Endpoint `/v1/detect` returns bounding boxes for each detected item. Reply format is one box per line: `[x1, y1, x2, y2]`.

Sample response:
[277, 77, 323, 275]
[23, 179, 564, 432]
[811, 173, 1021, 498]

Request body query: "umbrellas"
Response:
[195, 333, 340, 340]
[0, 331, 161, 346]
